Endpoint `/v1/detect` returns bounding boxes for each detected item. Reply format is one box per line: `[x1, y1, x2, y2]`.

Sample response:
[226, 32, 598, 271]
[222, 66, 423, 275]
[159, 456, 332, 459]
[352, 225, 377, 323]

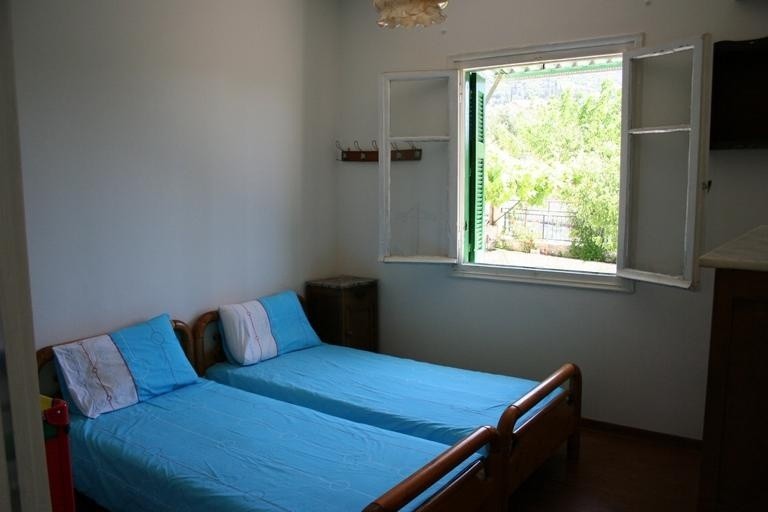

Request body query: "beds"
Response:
[193, 309, 582, 510]
[39, 319, 499, 510]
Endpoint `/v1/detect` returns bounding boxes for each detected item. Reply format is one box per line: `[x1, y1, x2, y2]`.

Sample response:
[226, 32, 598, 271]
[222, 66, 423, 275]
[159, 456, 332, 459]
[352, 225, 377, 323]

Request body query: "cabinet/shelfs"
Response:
[304, 275, 379, 352]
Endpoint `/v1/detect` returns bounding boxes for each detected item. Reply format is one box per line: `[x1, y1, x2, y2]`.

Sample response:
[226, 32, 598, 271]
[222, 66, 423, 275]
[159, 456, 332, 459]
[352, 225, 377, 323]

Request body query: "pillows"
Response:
[217, 289, 324, 368]
[49, 313, 199, 417]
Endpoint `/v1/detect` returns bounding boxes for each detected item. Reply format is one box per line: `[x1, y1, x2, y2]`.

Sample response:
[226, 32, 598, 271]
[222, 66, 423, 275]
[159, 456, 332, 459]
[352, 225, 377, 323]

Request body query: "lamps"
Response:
[372, 0, 450, 32]
